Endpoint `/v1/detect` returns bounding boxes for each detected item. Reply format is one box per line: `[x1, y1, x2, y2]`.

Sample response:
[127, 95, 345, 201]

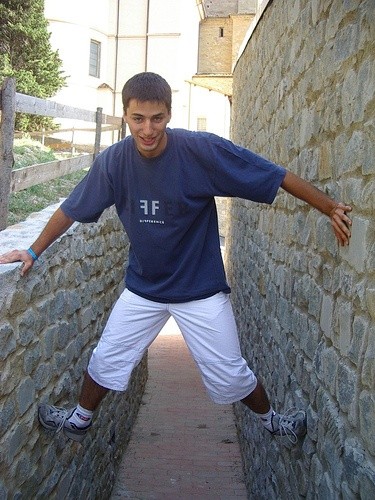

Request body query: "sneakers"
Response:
[38, 402, 92, 442]
[263, 409, 308, 437]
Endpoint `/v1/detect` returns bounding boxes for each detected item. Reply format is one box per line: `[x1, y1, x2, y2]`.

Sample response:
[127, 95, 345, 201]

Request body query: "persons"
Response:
[0, 70, 355, 446]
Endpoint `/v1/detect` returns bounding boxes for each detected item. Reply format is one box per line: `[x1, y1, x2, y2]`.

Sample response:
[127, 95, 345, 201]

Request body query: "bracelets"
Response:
[27, 248, 38, 260]
